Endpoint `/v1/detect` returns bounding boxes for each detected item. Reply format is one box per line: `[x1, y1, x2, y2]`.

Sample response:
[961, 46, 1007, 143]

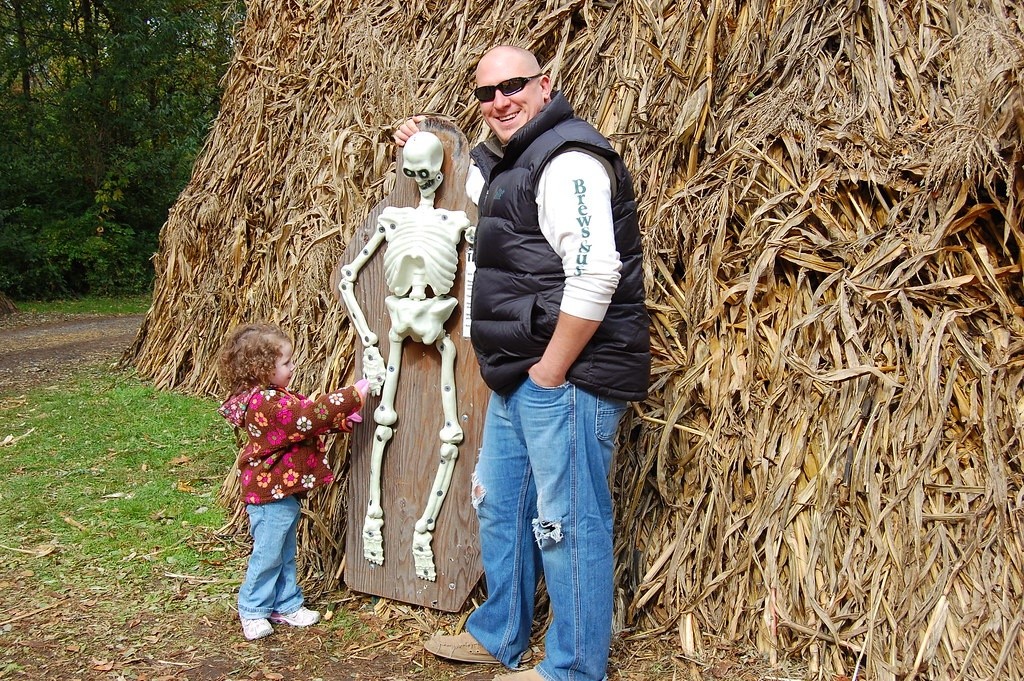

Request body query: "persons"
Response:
[393, 44, 650, 681]
[216, 323, 369, 640]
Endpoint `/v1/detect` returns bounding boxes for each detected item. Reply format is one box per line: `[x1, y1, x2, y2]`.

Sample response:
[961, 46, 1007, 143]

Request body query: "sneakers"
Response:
[491, 667, 545, 681]
[270, 604, 321, 627]
[423, 632, 532, 664]
[238, 605, 274, 640]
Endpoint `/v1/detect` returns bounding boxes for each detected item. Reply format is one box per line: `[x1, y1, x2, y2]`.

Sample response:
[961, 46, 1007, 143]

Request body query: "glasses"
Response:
[474, 73, 543, 103]
